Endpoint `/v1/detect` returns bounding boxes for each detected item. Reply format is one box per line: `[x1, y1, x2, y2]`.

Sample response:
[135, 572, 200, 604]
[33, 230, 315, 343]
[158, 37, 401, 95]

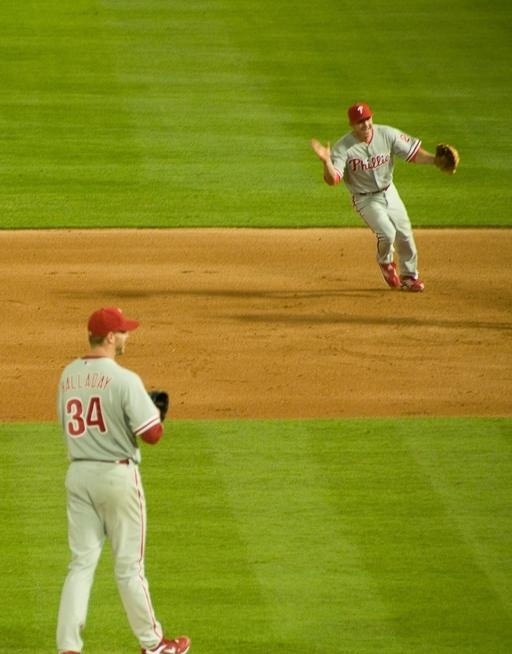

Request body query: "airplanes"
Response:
[147, 391, 167, 422]
[434, 144, 459, 174]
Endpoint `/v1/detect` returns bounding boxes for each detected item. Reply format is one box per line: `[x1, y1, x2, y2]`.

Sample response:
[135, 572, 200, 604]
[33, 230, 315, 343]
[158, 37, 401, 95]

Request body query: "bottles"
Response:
[349, 102, 372, 126]
[88, 308, 141, 336]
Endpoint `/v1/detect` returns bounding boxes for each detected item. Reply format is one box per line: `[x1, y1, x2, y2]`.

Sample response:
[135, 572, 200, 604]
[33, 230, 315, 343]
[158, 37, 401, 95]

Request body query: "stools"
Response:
[360, 184, 390, 195]
[120, 460, 129, 463]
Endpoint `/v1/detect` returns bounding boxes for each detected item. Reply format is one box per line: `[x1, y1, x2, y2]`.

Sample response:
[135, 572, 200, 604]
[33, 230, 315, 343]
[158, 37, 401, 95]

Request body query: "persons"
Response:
[54, 306, 191, 654]
[309, 103, 450, 292]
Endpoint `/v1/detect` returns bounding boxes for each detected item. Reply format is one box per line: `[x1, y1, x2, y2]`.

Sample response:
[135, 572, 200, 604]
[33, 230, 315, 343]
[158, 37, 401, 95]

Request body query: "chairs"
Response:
[400, 276, 425, 291]
[139, 636, 191, 654]
[379, 263, 400, 288]
